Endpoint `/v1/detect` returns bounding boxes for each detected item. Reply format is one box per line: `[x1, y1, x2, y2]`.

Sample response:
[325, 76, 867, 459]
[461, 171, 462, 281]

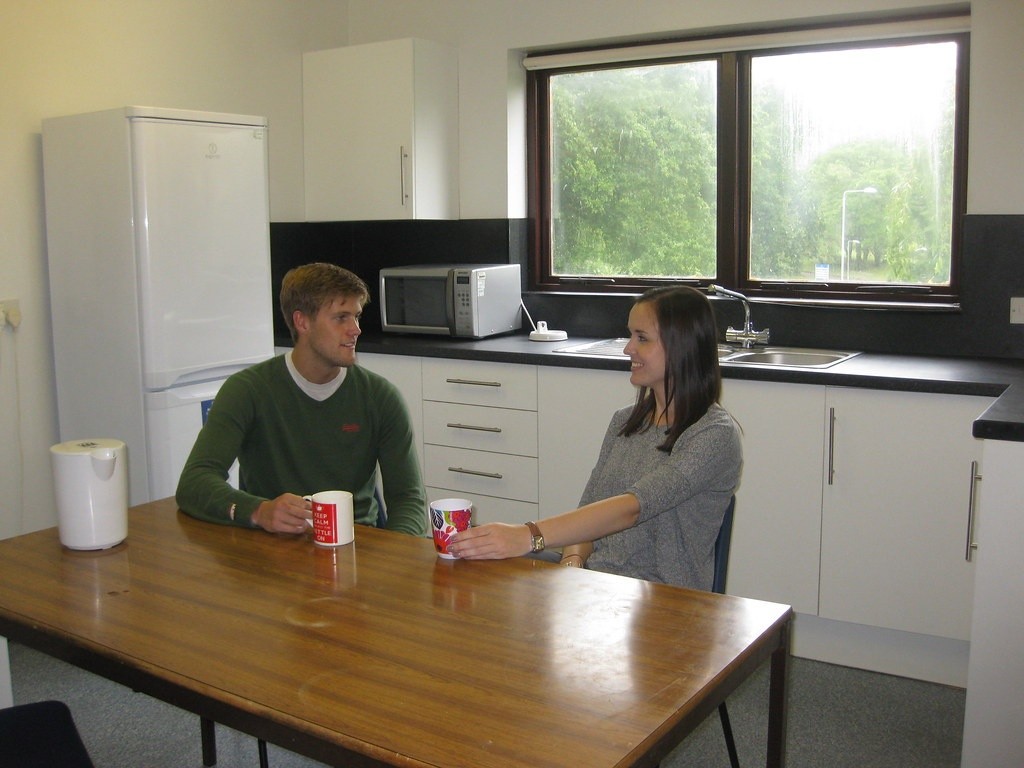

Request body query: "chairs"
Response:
[201, 396, 744, 767]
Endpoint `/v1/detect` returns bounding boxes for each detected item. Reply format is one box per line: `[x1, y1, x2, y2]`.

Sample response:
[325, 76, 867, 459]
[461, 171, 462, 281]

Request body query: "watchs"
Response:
[525, 521, 545, 554]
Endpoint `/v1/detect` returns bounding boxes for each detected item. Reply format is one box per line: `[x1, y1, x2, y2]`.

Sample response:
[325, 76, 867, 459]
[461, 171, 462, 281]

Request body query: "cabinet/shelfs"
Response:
[354, 353, 1002, 688]
[302, 36, 457, 221]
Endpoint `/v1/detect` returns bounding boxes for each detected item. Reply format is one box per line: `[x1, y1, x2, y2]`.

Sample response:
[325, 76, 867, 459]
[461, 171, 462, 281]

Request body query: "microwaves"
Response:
[379, 264, 522, 339]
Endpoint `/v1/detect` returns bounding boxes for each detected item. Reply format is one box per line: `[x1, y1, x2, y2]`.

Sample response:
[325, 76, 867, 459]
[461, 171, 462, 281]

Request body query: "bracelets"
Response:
[561, 554, 585, 568]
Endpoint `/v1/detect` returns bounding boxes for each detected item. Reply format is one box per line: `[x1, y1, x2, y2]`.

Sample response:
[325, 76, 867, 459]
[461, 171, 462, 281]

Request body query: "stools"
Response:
[1, 702, 94, 767]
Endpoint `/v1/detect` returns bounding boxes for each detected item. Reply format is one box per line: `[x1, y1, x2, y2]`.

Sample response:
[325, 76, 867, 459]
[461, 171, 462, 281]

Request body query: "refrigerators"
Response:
[41, 105, 276, 509]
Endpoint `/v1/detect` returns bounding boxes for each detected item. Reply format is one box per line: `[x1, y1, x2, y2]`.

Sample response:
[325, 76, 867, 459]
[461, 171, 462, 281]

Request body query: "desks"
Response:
[1, 494, 793, 768]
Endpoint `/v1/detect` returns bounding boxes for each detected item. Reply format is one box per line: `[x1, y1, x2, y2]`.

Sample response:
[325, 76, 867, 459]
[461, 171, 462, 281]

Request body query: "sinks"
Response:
[719, 345, 865, 369]
[717, 343, 749, 362]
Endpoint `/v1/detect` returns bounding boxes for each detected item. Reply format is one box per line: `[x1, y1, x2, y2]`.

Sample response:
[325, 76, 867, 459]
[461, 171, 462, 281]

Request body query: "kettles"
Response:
[50, 438, 128, 550]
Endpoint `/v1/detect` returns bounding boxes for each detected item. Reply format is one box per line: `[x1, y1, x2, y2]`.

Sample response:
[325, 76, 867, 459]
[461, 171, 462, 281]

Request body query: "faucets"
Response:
[707, 283, 772, 349]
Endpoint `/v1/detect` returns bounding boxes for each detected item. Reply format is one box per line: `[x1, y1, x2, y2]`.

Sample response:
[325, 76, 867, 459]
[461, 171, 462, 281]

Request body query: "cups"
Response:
[430, 499, 472, 559]
[302, 491, 354, 546]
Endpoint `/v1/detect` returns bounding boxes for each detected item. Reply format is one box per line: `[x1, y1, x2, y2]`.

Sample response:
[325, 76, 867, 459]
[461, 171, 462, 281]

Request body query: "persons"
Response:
[446, 286, 744, 592]
[175, 263, 429, 537]
